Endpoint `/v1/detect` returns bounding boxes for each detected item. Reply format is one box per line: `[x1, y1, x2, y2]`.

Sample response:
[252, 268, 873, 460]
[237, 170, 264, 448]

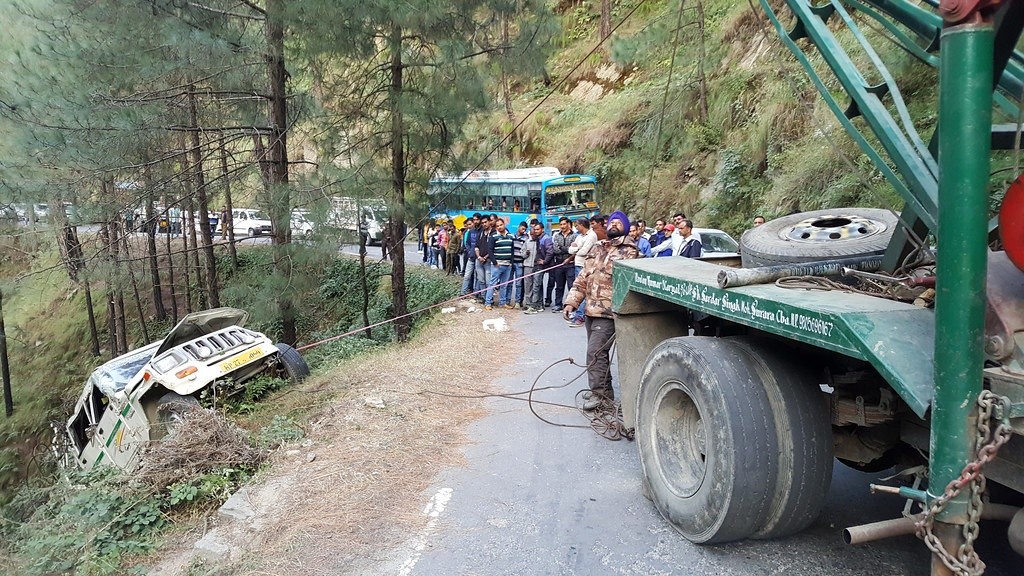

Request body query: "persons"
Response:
[359, 215, 369, 256]
[562, 211, 642, 411]
[753, 217, 765, 227]
[676, 220, 702, 258]
[418, 212, 609, 327]
[482, 198, 520, 212]
[629, 214, 700, 259]
[208, 210, 219, 240]
[381, 222, 394, 260]
[221, 206, 228, 240]
[125, 208, 133, 232]
[170, 205, 181, 239]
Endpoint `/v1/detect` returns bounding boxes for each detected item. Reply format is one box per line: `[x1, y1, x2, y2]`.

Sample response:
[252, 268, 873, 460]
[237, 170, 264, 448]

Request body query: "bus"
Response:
[425, 166, 600, 238]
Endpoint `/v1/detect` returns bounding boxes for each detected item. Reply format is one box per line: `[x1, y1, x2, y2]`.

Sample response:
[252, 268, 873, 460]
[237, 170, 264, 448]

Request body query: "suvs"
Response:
[50, 306, 312, 491]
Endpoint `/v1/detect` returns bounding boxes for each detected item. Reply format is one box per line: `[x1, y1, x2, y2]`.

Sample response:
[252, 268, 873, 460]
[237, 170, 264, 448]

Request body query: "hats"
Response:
[605, 210, 630, 234]
[663, 223, 675, 231]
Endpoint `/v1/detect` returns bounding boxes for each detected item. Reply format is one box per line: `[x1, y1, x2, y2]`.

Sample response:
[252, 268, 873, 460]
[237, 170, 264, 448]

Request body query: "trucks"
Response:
[611, 1, 1024, 575]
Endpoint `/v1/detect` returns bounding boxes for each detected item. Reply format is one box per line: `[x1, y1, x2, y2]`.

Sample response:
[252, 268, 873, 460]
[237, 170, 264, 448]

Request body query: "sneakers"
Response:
[523, 307, 537, 313]
[570, 314, 584, 327]
[537, 306, 544, 312]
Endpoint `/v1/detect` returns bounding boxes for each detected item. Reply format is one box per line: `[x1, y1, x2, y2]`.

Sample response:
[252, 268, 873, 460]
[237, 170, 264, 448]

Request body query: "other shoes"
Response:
[583, 390, 593, 399]
[523, 306, 529, 310]
[458, 294, 466, 301]
[552, 305, 563, 311]
[499, 304, 513, 309]
[515, 303, 520, 309]
[545, 302, 550, 307]
[485, 305, 492, 310]
[582, 392, 602, 409]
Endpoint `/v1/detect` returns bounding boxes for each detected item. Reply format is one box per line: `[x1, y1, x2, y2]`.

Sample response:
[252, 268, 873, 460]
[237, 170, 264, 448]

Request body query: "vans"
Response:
[325, 195, 391, 247]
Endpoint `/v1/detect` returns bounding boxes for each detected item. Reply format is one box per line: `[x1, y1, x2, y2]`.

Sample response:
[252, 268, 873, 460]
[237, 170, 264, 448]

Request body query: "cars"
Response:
[117, 204, 320, 242]
[0, 200, 83, 223]
[672, 227, 740, 257]
